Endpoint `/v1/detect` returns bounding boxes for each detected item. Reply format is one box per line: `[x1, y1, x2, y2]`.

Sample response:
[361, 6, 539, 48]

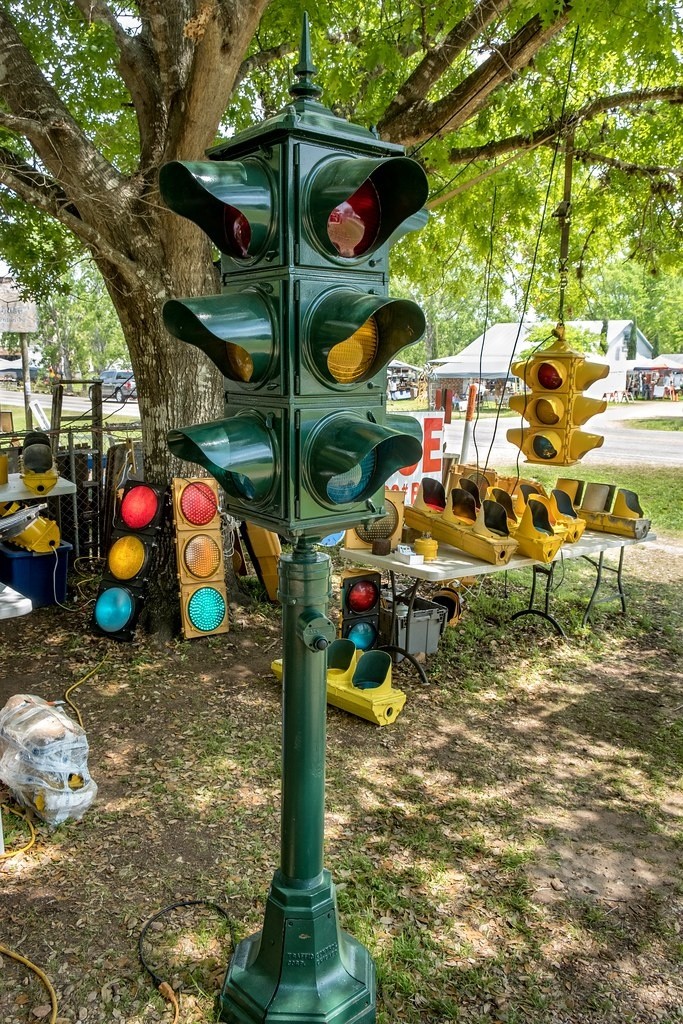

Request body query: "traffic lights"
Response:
[430, 586, 462, 628]
[289, 126, 423, 536]
[506, 351, 534, 464]
[89, 478, 167, 642]
[528, 351, 570, 469]
[270, 637, 406, 728]
[159, 129, 291, 544]
[17, 432, 60, 497]
[169, 474, 232, 640]
[337, 567, 382, 656]
[0, 500, 61, 552]
[564, 356, 610, 469]
[553, 476, 652, 540]
[403, 464, 588, 567]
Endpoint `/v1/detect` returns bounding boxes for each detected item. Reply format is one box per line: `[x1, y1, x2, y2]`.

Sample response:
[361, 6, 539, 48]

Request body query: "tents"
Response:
[431, 319, 683, 403]
[387, 360, 421, 373]
[626, 358, 683, 391]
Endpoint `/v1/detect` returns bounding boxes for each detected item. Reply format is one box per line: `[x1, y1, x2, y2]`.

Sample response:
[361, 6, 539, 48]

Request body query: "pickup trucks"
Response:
[88, 369, 137, 404]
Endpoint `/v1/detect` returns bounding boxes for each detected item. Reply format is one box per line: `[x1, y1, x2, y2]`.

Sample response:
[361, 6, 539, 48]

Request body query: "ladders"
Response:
[49, 379, 103, 559]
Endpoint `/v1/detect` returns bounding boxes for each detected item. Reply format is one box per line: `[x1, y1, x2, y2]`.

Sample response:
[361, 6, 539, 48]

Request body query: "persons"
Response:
[387, 371, 682, 412]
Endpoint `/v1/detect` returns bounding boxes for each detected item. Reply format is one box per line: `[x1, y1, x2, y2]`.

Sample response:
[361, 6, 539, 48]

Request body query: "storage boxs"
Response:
[376, 586, 449, 665]
[0, 538, 73, 611]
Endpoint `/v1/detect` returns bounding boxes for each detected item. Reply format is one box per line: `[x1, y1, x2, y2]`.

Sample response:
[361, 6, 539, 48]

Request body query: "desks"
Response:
[0, 473, 80, 570]
[341, 527, 657, 684]
